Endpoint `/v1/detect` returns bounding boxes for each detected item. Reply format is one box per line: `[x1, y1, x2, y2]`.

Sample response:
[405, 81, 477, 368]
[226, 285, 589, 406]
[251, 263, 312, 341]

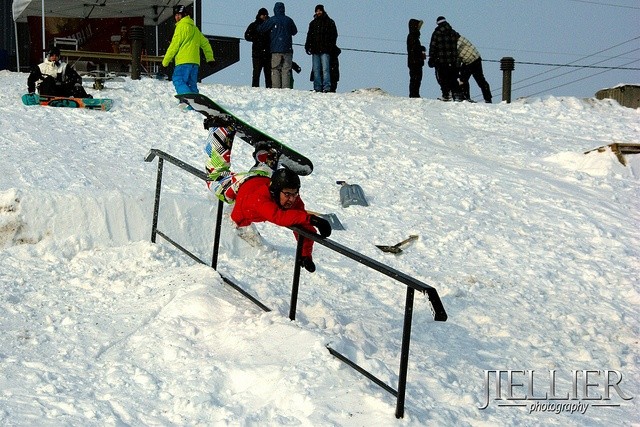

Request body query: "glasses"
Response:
[281, 191, 299, 198]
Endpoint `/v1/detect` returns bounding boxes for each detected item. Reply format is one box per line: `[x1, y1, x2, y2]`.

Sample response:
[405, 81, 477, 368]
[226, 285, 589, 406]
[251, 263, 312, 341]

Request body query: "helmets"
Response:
[270, 169, 300, 193]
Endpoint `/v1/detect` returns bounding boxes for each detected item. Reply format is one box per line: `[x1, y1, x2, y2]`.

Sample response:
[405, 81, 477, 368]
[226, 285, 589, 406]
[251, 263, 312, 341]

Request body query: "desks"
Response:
[59, 49, 167, 78]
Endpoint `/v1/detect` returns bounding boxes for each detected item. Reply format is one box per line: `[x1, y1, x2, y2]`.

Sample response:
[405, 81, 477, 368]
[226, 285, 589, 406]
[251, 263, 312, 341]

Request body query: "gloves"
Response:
[301, 256, 315, 272]
[310, 215, 331, 238]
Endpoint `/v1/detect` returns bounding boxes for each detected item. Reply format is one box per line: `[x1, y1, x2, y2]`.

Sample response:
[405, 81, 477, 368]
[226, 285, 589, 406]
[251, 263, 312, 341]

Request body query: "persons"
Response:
[245, 7, 273, 89]
[310, 43, 340, 90]
[429, 16, 463, 101]
[452, 31, 494, 104]
[260, 5, 298, 92]
[202, 113, 331, 273]
[406, 18, 426, 98]
[28, 46, 93, 98]
[305, 3, 337, 93]
[163, 4, 214, 110]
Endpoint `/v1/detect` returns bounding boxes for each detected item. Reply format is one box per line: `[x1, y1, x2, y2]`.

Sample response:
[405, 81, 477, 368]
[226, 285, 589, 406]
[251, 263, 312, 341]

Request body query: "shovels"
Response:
[336, 181, 369, 208]
[375, 235, 418, 253]
[306, 211, 345, 229]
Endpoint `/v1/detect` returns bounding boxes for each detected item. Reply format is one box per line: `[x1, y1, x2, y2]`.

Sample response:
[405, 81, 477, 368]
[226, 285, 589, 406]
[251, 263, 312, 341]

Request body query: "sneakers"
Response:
[247, 135, 273, 147]
[75, 93, 91, 98]
[204, 117, 238, 132]
[39, 83, 49, 95]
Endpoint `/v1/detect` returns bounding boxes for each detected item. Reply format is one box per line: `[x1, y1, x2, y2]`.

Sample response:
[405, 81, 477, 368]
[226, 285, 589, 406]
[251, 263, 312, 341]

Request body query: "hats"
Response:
[315, 5, 324, 12]
[50, 48, 59, 55]
[437, 17, 446, 23]
[173, 5, 188, 13]
[258, 8, 268, 15]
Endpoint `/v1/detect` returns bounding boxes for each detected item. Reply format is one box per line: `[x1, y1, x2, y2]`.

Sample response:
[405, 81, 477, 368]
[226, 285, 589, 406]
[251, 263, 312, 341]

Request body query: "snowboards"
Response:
[174, 93, 314, 176]
[436, 97, 477, 102]
[21, 93, 113, 111]
